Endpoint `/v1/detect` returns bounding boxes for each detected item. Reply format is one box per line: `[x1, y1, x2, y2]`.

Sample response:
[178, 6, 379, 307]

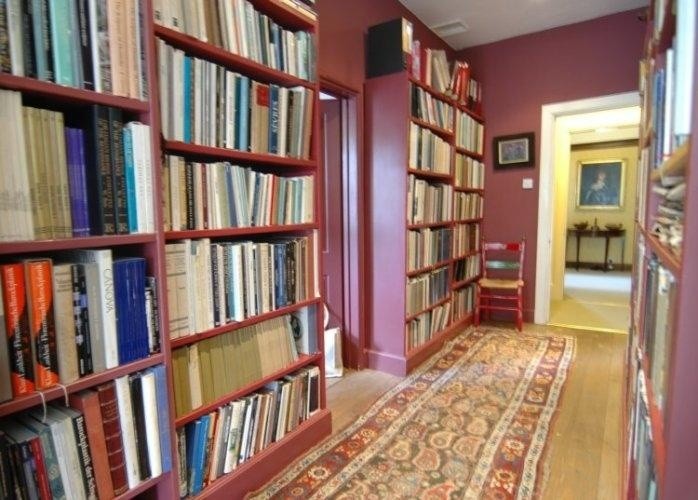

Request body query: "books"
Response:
[152, 2, 319, 497]
[623, 0, 698, 500]
[0, 0, 174, 499]
[366, 18, 486, 354]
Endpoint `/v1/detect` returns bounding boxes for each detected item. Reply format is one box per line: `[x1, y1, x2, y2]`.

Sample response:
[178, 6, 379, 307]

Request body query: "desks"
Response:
[567, 229, 624, 273]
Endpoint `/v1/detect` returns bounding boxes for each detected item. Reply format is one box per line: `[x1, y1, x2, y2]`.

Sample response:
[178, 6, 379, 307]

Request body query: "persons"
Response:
[584, 170, 617, 205]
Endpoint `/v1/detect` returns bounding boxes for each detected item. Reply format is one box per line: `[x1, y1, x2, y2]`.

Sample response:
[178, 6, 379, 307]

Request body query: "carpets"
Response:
[240, 323, 577, 499]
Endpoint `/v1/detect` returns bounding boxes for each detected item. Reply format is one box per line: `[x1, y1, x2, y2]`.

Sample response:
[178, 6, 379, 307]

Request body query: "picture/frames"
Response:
[576, 159, 625, 210]
[493, 132, 535, 171]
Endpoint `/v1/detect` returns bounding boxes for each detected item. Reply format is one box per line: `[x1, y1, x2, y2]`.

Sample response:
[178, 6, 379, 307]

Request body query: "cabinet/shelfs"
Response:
[363, 71, 486, 376]
[624, 1, 698, 500]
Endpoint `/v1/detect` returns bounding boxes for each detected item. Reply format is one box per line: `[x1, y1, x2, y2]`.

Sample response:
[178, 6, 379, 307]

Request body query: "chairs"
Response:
[476, 237, 527, 332]
